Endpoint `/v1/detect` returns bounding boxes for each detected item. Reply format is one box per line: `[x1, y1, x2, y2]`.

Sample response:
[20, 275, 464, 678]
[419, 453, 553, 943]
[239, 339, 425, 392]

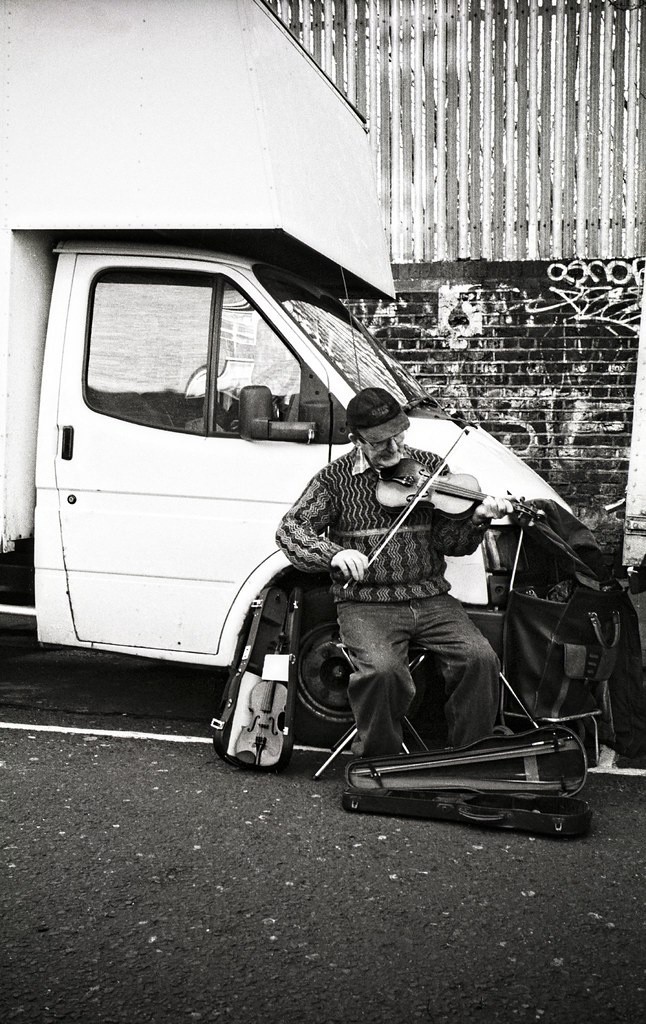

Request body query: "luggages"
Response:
[492, 499, 646, 766]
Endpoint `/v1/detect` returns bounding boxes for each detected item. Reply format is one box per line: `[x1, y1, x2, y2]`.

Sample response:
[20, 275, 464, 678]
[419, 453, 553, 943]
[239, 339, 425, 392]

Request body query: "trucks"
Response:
[3, 0, 578, 752]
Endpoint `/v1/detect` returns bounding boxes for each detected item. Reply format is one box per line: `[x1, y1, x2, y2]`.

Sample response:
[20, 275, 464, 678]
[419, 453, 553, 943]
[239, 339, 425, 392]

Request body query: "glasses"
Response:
[354, 432, 405, 452]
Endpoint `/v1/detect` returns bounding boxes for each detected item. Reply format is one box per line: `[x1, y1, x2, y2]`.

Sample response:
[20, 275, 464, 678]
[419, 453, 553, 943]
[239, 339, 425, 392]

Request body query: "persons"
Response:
[275, 388, 515, 757]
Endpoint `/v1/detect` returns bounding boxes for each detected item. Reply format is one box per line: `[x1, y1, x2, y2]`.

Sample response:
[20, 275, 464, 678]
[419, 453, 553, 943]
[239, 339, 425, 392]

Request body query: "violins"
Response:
[374, 456, 548, 528]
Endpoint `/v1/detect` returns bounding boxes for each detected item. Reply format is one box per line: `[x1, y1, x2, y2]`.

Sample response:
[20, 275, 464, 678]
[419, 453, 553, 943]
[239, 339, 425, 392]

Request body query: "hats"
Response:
[345, 388, 410, 443]
